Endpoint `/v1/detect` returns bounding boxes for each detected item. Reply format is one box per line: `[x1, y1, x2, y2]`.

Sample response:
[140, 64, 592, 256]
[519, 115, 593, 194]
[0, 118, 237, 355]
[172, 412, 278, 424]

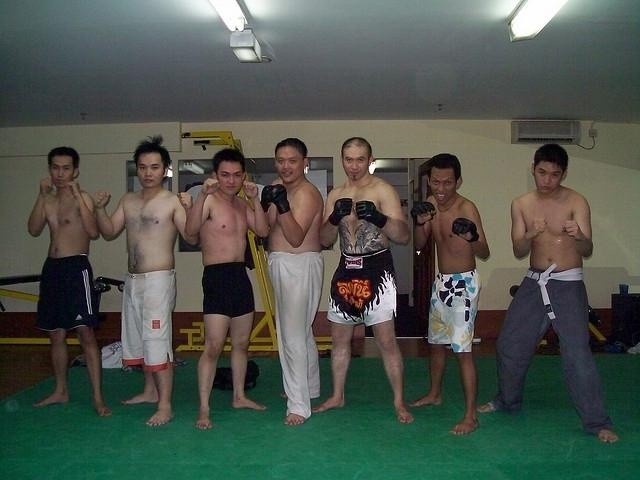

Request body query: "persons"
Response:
[93, 137, 198, 426]
[408, 153, 489, 435]
[186, 150, 268, 430]
[476, 145, 620, 444]
[311, 138, 414, 424]
[27, 147, 112, 417]
[261, 138, 324, 427]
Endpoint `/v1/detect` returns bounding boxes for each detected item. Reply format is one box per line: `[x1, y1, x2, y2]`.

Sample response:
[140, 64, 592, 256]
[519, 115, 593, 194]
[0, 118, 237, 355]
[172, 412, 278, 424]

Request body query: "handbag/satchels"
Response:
[212, 361, 259, 391]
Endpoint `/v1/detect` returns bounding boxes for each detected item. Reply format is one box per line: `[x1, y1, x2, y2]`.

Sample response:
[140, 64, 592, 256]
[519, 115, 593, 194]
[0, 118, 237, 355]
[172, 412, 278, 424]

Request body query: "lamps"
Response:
[228, 29, 262, 64]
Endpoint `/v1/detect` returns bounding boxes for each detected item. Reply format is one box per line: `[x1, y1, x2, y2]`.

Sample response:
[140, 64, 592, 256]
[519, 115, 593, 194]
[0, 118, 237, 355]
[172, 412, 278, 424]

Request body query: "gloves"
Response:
[260, 185, 274, 212]
[270, 185, 290, 215]
[355, 201, 386, 228]
[327, 199, 351, 225]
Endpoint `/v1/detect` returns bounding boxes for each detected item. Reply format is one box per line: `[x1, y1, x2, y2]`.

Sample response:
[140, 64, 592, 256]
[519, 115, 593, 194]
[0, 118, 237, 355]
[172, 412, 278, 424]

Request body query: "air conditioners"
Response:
[510, 120, 582, 146]
